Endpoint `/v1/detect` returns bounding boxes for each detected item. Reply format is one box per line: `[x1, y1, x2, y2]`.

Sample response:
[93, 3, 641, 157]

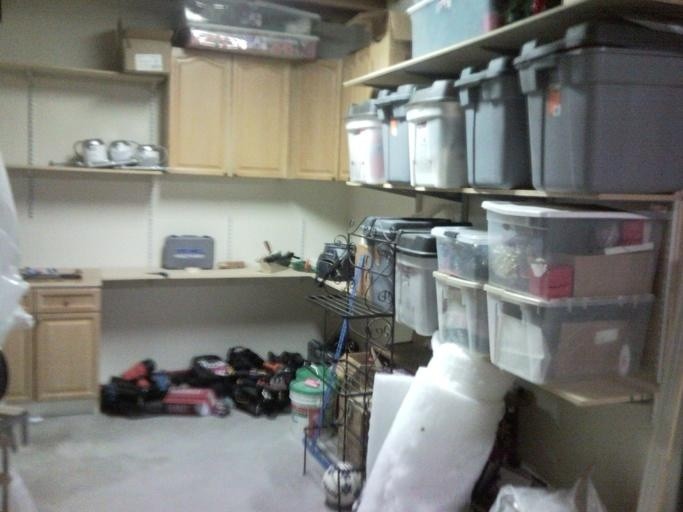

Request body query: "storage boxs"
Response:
[344, 98, 386, 188]
[483, 284, 656, 391]
[481, 199, 674, 302]
[341, 8, 413, 83]
[336, 352, 376, 392]
[117, 15, 174, 76]
[337, 391, 371, 440]
[177, 0, 323, 36]
[404, 0, 500, 61]
[166, 22, 320, 62]
[513, 22, 683, 195]
[406, 78, 467, 192]
[347, 215, 473, 338]
[430, 226, 489, 284]
[374, 83, 416, 189]
[334, 425, 364, 470]
[453, 55, 533, 190]
[433, 270, 489, 356]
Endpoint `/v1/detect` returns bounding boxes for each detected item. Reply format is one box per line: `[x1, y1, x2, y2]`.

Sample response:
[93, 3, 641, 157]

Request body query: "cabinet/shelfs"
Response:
[289, 58, 340, 180]
[33, 283, 101, 417]
[341, 35, 389, 179]
[0, 59, 314, 283]
[229, 57, 289, 177]
[0, 284, 32, 418]
[166, 45, 233, 176]
[317, 0, 683, 408]
